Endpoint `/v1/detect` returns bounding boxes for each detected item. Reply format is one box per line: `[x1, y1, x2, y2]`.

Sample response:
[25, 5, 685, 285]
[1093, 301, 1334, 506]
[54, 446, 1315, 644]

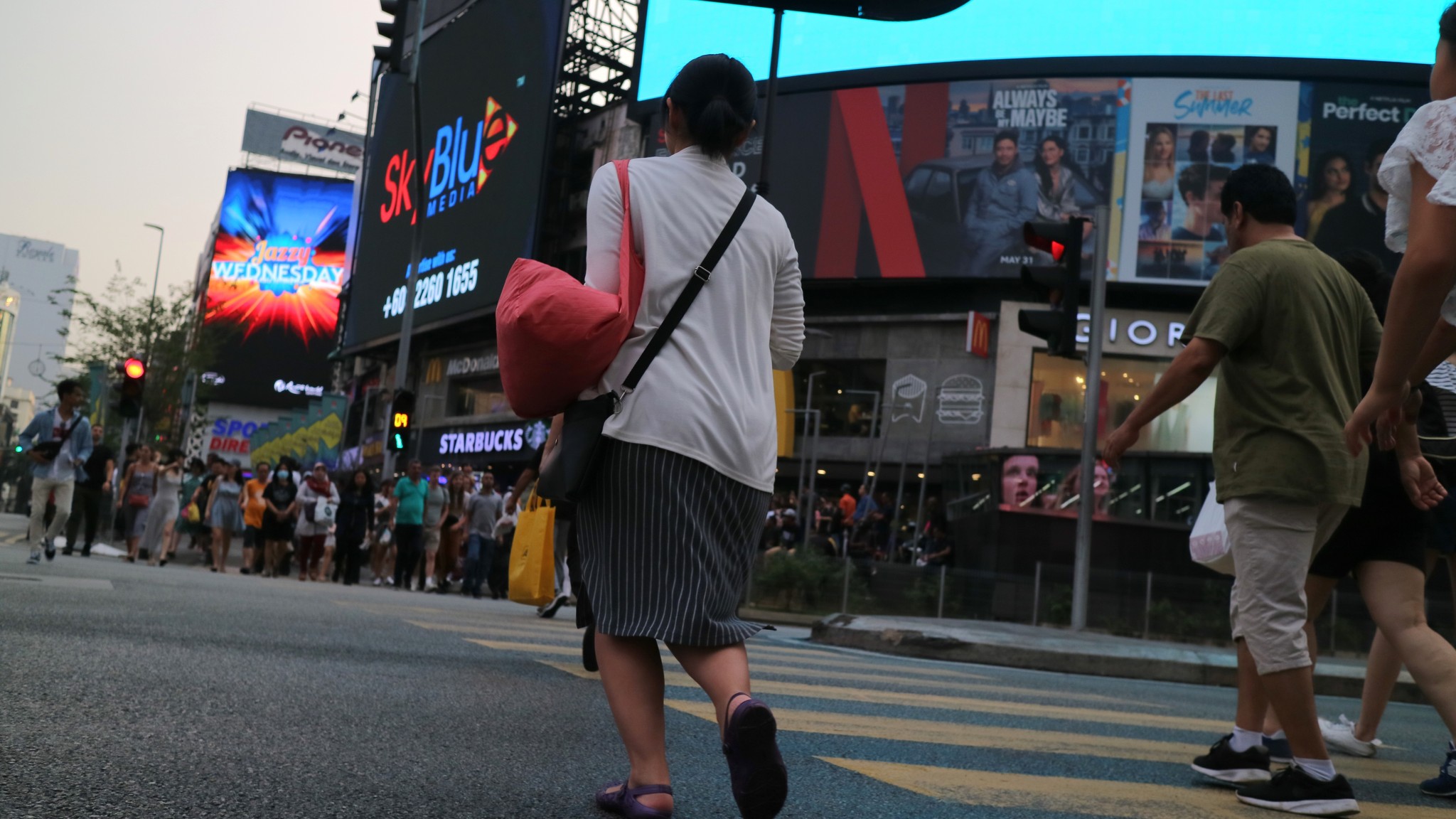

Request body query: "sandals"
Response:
[721, 692, 787, 819]
[595, 776, 675, 819]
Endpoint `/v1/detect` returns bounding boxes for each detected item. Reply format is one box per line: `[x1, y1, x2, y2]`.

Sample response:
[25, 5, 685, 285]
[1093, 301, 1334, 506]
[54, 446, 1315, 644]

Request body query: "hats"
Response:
[313, 462, 326, 471]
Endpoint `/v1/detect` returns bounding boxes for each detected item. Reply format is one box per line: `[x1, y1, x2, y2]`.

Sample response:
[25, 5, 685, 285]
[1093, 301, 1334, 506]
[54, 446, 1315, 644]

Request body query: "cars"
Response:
[902, 153, 999, 233]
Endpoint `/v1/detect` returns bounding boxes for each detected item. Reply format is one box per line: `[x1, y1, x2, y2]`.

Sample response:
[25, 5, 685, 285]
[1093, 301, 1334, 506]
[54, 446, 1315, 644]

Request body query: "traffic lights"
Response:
[374, 0, 426, 81]
[1015, 214, 1087, 356]
[388, 388, 418, 453]
[119, 357, 147, 415]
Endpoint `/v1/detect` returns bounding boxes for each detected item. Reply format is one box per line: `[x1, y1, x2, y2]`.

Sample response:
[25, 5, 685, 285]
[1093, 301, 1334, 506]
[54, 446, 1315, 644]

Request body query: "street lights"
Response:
[134, 223, 166, 442]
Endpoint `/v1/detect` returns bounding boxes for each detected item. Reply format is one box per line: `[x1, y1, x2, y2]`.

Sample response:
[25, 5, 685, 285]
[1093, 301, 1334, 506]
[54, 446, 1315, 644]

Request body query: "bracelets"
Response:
[106, 480, 112, 484]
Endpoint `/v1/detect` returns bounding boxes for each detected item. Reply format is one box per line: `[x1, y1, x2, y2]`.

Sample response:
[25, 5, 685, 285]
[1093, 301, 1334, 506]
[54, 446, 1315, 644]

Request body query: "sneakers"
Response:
[1191, 733, 1272, 783]
[1419, 741, 1456, 796]
[1317, 714, 1380, 757]
[1236, 762, 1361, 815]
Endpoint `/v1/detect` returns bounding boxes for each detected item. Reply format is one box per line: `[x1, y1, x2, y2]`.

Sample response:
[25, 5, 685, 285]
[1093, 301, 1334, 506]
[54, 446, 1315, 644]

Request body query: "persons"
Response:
[1026, 135, 1094, 268]
[756, 475, 957, 567]
[962, 130, 1039, 277]
[1090, 163, 1451, 814]
[1256, 0, 1455, 799]
[117, 441, 573, 618]
[20, 377, 95, 563]
[1314, 151, 1409, 331]
[1135, 123, 1275, 278]
[1305, 150, 1356, 245]
[1001, 454, 1056, 511]
[1054, 458, 1113, 516]
[517, 50, 807, 818]
[63, 422, 115, 556]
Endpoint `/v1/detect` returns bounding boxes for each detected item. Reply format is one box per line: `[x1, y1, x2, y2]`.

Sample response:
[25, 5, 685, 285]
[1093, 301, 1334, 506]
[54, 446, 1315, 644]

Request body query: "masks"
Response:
[276, 470, 289, 479]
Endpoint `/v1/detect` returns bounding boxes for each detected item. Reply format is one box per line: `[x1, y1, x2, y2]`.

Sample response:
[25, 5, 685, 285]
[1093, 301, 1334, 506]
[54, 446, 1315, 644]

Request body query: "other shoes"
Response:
[26, 553, 41, 565]
[535, 592, 570, 618]
[1261, 731, 1294, 763]
[43, 536, 55, 561]
[62, 548, 507, 601]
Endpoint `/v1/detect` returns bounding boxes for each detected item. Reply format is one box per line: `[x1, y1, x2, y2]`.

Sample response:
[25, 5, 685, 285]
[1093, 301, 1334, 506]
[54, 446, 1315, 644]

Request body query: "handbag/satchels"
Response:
[494, 153, 651, 418]
[539, 394, 619, 498]
[30, 411, 86, 461]
[313, 496, 339, 528]
[508, 479, 556, 606]
[1188, 481, 1236, 575]
